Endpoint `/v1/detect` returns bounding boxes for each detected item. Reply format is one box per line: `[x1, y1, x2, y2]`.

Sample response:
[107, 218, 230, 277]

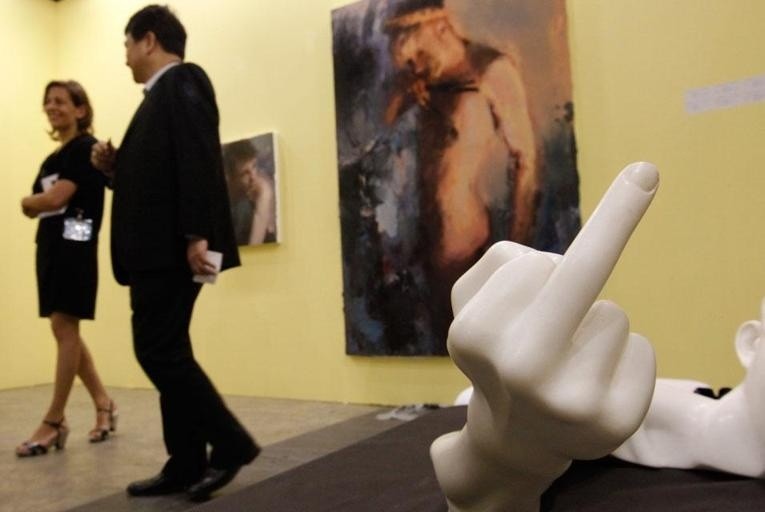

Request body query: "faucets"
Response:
[14, 418, 70, 458]
[88, 401, 118, 443]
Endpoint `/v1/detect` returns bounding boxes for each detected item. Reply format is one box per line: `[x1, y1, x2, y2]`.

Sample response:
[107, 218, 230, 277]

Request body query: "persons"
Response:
[14, 80, 118, 458]
[219, 139, 273, 245]
[429, 159, 765, 510]
[88, 3, 263, 504]
[379, 1, 538, 331]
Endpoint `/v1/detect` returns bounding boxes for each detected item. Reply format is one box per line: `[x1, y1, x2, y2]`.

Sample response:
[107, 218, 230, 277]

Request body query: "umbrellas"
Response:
[188, 432, 261, 498]
[127, 457, 210, 497]
